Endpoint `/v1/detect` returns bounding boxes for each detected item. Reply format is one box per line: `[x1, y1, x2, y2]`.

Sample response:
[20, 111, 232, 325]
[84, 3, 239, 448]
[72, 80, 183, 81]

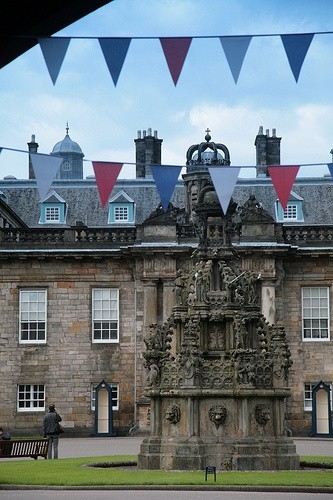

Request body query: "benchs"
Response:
[0, 437, 50, 461]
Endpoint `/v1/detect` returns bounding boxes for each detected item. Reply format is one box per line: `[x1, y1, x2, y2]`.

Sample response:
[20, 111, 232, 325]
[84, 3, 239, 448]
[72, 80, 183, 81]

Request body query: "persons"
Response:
[43, 404, 63, 459]
[244, 194, 261, 208]
[0, 428, 13, 457]
